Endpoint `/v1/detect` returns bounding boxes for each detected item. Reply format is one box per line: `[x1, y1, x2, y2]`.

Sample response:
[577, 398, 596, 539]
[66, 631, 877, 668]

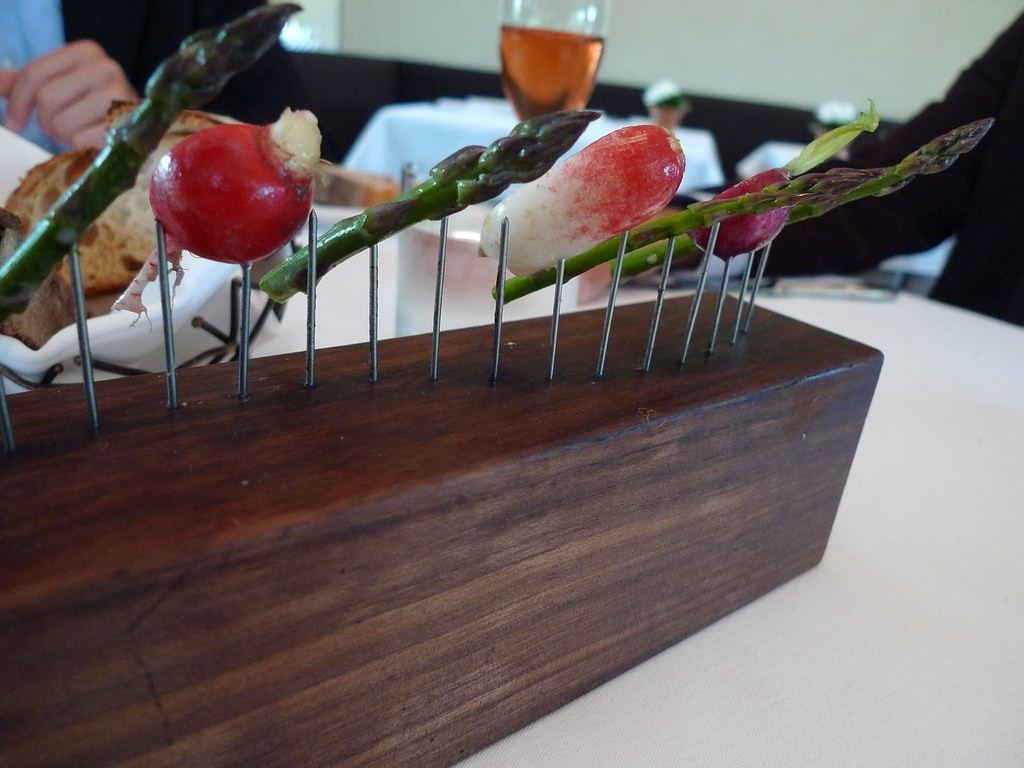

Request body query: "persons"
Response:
[1, 0, 307, 157]
[577, 12, 1024, 324]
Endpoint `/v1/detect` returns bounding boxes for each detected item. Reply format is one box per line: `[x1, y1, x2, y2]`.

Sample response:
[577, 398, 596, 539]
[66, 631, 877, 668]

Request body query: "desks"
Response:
[740, 137, 958, 301]
[0, 206, 1023, 768]
[352, 100, 725, 209]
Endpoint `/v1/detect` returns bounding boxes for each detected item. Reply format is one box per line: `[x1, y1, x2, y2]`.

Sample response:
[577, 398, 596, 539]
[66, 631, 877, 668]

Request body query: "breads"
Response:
[1, 97, 395, 348]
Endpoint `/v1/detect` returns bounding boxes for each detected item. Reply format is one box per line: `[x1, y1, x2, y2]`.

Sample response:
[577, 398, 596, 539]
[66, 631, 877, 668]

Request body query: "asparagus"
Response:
[490, 163, 911, 303]
[259, 106, 604, 303]
[1, 1, 305, 321]
[609, 117, 995, 280]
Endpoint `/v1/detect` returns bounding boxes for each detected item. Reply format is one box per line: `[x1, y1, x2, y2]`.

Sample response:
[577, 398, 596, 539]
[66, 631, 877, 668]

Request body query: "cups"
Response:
[497, 0, 613, 120]
[391, 159, 582, 338]
